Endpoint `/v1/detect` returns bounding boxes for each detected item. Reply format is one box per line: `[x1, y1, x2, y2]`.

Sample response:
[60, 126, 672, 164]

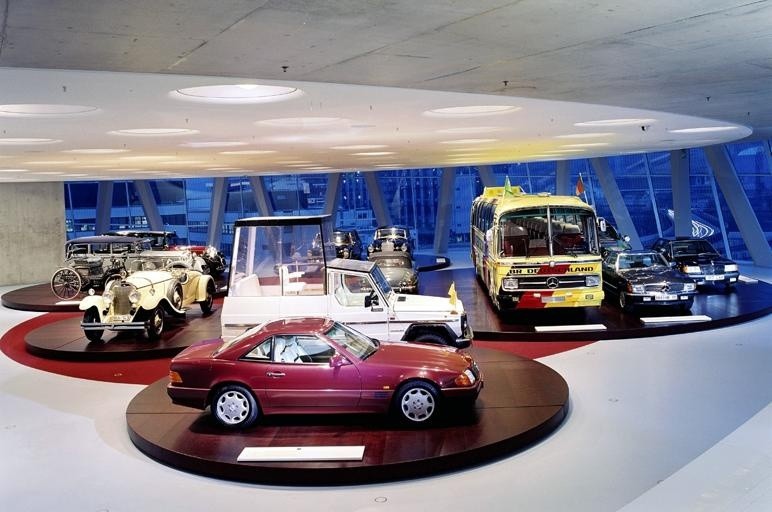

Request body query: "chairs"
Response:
[498, 216, 585, 257]
[250, 333, 314, 363]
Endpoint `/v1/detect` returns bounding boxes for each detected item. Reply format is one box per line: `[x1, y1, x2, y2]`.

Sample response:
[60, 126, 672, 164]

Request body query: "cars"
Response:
[599, 232, 740, 311]
[166, 317, 486, 427]
[50, 230, 227, 343]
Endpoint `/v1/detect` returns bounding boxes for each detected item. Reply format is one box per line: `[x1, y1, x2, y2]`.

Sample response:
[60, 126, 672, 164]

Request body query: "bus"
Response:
[470, 186, 607, 312]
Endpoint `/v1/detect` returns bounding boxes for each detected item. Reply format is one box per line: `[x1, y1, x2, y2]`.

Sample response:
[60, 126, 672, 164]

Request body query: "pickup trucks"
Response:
[220, 214, 474, 349]
